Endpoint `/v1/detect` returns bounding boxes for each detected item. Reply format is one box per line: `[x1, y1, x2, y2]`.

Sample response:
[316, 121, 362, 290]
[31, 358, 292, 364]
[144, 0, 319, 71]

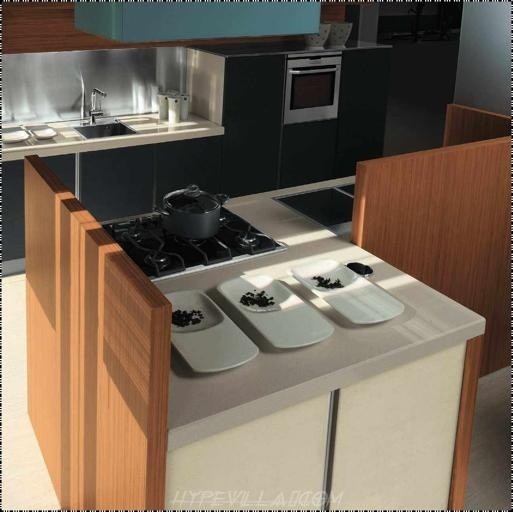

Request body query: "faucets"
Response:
[88, 88, 107, 125]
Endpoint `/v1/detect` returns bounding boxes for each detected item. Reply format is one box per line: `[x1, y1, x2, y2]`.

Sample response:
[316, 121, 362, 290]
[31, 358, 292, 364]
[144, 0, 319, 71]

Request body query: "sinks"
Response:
[72, 120, 137, 141]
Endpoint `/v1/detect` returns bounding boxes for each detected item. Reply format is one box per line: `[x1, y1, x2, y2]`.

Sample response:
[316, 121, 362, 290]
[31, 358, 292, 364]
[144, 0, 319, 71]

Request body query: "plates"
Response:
[216, 274, 335, 349]
[26, 123, 58, 139]
[291, 259, 405, 324]
[164, 291, 260, 374]
[1, 122, 28, 143]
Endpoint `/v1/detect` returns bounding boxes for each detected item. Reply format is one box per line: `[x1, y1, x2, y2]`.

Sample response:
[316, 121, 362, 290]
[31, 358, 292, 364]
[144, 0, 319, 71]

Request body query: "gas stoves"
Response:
[111, 215, 283, 280]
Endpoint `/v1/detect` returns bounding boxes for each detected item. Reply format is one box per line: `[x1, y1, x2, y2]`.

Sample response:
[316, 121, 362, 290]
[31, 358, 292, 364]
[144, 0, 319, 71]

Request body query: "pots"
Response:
[153, 184, 228, 239]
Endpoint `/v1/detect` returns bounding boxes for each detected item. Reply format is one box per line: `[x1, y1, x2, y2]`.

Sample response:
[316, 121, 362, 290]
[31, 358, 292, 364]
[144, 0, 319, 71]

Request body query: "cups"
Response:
[167, 95, 181, 124]
[179, 94, 189, 121]
[157, 94, 171, 121]
[166, 89, 179, 96]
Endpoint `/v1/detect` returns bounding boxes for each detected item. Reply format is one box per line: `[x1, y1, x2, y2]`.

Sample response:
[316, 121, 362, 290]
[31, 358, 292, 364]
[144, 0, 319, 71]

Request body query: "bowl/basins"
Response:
[304, 24, 331, 50]
[325, 21, 353, 49]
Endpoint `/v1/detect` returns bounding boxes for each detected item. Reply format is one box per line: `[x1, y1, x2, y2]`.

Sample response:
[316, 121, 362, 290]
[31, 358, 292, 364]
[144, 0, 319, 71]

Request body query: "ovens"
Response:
[283, 51, 343, 125]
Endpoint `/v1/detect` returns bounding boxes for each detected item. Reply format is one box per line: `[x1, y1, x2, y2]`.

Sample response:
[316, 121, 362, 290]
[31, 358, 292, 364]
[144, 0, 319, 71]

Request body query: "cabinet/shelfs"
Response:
[219, 54, 286, 198]
[1, 153, 77, 263]
[164, 340, 467, 512]
[335, 49, 392, 216]
[279, 122, 335, 224]
[77, 136, 222, 225]
